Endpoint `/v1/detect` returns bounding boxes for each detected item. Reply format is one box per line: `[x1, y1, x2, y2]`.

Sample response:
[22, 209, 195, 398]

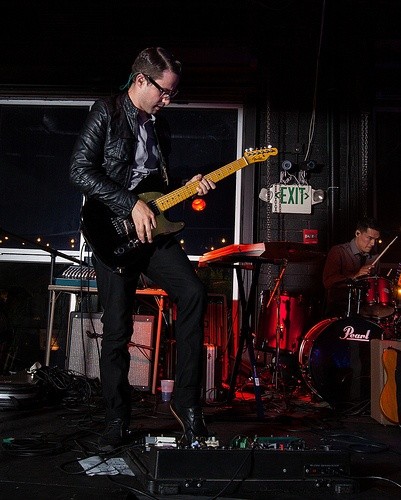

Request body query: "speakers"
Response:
[63, 311, 155, 395]
[369, 338, 401, 426]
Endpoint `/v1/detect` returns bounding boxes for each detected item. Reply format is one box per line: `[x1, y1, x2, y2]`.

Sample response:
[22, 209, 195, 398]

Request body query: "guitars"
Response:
[80, 144, 279, 274]
[379, 262, 401, 423]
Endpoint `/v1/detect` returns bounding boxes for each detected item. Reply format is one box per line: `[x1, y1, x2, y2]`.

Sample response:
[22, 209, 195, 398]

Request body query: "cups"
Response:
[161, 379, 174, 401]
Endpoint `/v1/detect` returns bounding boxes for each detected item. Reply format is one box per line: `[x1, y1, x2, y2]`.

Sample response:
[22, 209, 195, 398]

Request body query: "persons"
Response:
[322, 216, 384, 316]
[70, 45, 217, 441]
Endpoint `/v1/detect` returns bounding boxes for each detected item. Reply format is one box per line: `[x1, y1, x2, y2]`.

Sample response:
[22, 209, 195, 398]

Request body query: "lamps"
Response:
[259, 185, 324, 214]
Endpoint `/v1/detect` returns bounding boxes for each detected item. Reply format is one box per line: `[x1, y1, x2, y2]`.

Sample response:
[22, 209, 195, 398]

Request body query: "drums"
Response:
[298, 314, 393, 410]
[351, 273, 396, 319]
[252, 289, 324, 357]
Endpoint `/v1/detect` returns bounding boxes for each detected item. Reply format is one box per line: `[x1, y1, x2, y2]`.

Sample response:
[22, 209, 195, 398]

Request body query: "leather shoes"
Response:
[106, 418, 135, 443]
[172, 401, 208, 439]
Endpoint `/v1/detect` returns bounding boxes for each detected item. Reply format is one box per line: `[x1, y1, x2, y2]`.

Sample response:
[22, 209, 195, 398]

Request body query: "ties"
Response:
[139, 112, 159, 169]
[358, 253, 368, 268]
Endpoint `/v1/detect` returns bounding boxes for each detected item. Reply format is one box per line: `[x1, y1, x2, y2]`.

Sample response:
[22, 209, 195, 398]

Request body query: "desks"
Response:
[46, 285, 168, 394]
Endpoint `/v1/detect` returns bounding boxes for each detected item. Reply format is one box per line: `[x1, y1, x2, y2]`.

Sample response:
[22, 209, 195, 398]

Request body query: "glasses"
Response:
[135, 73, 178, 98]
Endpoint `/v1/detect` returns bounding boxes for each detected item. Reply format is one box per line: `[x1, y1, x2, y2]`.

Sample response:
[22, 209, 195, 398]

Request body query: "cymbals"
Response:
[258, 240, 327, 262]
